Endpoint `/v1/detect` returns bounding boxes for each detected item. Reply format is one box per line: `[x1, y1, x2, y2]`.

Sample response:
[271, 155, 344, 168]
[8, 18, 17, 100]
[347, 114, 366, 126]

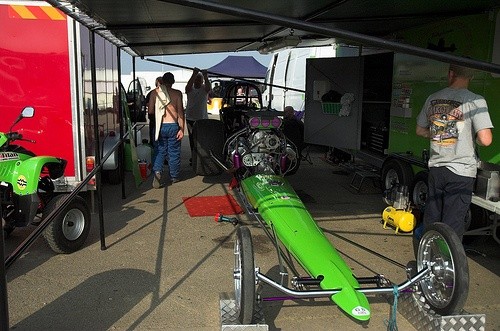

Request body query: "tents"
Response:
[206, 55, 268, 79]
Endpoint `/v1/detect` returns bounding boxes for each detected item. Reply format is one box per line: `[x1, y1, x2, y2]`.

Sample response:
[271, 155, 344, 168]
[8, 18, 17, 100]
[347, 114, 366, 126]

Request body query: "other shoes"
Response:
[152, 172, 161, 189]
[170, 179, 179, 184]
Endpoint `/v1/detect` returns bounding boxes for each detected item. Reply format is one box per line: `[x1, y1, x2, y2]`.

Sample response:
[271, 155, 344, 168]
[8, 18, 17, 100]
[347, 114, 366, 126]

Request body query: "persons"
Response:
[415, 56, 494, 243]
[213, 83, 221, 90]
[148, 72, 185, 188]
[284, 106, 304, 153]
[185, 67, 212, 162]
[145, 77, 169, 165]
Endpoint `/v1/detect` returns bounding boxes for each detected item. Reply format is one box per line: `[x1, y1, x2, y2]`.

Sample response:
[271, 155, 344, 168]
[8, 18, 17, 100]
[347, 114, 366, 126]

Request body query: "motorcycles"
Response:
[0, 105, 92, 262]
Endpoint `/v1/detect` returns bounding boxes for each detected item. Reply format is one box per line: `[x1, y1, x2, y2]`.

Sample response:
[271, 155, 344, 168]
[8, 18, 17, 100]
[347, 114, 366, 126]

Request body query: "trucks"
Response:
[258, 43, 388, 123]
[0, 0, 119, 191]
[303, 40, 500, 245]
